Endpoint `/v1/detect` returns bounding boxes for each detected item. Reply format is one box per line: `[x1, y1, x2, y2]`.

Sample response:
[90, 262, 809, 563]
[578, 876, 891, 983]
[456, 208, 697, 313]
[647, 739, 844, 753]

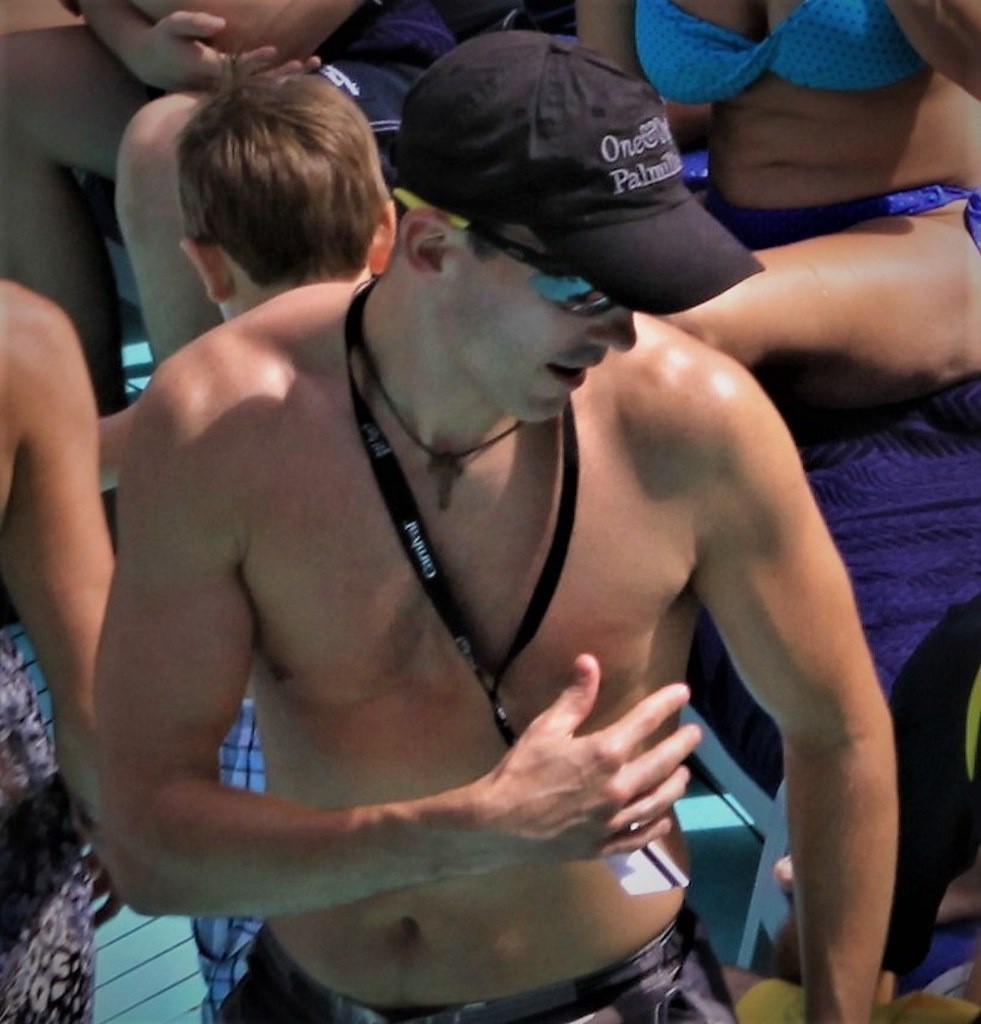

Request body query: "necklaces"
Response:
[348, 262, 540, 510]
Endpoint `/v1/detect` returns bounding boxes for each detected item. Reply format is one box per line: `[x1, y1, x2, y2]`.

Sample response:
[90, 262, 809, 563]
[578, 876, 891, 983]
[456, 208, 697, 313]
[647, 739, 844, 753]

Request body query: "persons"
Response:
[0, 250, 166, 1021]
[93, 30, 903, 1024]
[700, 586, 981, 1022]
[566, 1, 980, 997]
[0, 0, 550, 548]
[143, 65, 435, 1024]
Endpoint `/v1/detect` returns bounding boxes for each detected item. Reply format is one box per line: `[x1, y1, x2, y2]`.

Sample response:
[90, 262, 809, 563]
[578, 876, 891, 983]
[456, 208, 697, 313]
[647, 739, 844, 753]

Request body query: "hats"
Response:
[391, 31, 765, 315]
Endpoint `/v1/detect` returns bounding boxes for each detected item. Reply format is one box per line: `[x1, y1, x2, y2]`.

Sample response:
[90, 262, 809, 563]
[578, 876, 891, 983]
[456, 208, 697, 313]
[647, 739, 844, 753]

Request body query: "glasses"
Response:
[391, 184, 615, 313]
